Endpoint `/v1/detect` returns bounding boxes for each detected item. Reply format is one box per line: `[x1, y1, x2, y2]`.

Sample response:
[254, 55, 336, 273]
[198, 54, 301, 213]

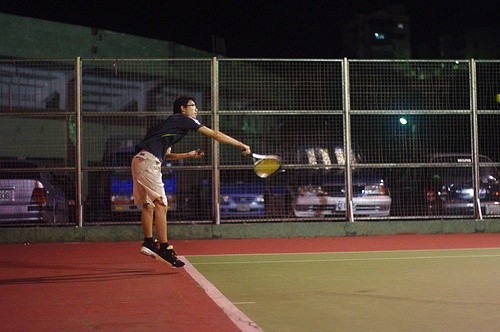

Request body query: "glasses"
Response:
[185, 104, 196, 108]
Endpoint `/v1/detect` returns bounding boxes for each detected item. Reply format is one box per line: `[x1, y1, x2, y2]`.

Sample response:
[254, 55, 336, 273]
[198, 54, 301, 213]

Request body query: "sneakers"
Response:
[140, 241, 157, 258]
[155, 245, 186, 269]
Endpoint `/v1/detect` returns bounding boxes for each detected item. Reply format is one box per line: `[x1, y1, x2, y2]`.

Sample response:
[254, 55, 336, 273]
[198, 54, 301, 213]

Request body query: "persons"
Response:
[130, 96, 251, 270]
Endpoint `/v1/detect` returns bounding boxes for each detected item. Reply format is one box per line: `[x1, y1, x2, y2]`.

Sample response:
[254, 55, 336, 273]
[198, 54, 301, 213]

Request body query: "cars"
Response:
[286, 162, 391, 221]
[405, 152, 500, 218]
[0, 159, 71, 226]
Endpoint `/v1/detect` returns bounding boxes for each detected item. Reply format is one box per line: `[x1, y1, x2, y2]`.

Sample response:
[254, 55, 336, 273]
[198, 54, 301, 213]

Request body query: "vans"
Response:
[199, 161, 287, 219]
[97, 134, 177, 219]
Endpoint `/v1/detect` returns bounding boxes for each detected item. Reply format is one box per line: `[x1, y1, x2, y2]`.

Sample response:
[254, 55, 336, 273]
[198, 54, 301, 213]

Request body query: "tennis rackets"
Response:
[240, 147, 282, 179]
